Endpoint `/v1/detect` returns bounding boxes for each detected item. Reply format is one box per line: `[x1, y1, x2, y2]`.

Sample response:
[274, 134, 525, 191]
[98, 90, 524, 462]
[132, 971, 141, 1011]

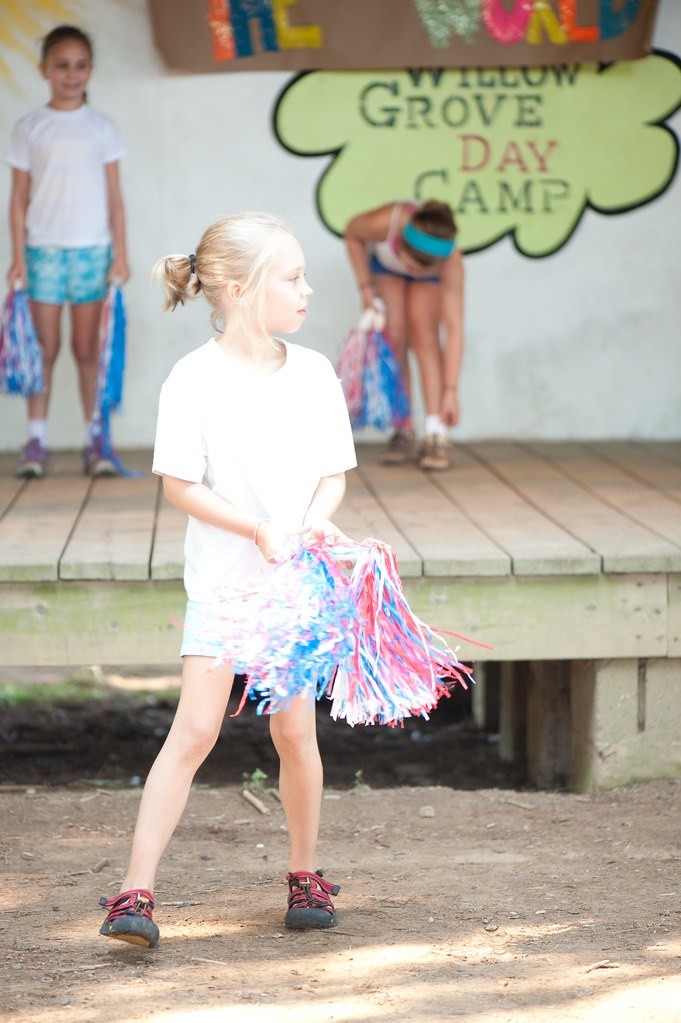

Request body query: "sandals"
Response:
[99, 889, 159, 949]
[286, 869, 341, 929]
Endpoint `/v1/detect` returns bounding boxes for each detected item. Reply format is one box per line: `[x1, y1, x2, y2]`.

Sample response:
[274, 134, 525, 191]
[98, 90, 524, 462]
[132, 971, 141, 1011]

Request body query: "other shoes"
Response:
[418, 433, 453, 471]
[15, 440, 48, 477]
[82, 443, 119, 477]
[381, 430, 415, 465]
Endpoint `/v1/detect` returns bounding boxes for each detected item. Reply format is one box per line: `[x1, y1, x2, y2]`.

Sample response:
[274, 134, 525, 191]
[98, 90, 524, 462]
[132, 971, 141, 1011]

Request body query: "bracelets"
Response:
[444, 385, 457, 391]
[253, 518, 273, 546]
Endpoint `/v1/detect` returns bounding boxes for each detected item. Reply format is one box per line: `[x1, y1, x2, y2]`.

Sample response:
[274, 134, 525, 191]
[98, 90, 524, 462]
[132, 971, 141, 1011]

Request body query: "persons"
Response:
[345, 201, 464, 471]
[100, 212, 359, 948]
[4, 23, 131, 476]
